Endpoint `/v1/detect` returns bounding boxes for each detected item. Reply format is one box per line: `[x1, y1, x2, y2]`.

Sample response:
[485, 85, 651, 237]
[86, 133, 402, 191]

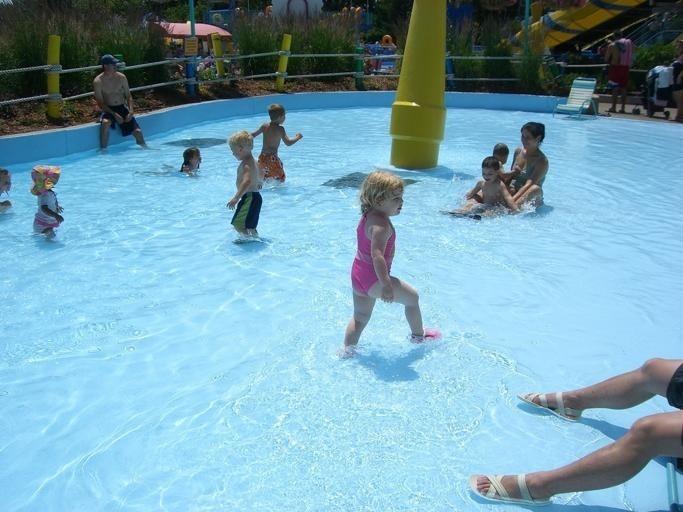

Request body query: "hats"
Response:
[30, 165, 61, 196]
[103, 54, 119, 65]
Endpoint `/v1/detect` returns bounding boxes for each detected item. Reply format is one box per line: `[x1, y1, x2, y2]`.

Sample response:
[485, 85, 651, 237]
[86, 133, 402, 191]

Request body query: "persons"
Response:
[493, 142, 521, 197]
[340, 170, 441, 358]
[603, 30, 632, 113]
[29, 165, 63, 242]
[94, 54, 144, 151]
[226, 131, 266, 244]
[447, 123, 550, 220]
[0, 169, 12, 214]
[251, 104, 302, 184]
[180, 148, 203, 173]
[468, 157, 521, 214]
[671, 41, 683, 124]
[470, 357, 683, 504]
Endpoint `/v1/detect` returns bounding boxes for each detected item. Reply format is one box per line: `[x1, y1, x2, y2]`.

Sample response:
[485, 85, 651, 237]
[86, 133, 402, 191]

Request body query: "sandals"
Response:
[605, 107, 617, 112]
[519, 390, 581, 422]
[472, 473, 550, 507]
[619, 109, 626, 113]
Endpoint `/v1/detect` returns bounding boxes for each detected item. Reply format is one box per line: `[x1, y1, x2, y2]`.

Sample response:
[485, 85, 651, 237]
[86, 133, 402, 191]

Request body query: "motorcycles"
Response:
[363, 41, 385, 75]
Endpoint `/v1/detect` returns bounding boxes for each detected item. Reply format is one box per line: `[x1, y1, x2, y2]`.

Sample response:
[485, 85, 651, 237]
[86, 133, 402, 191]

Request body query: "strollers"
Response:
[646, 61, 683, 120]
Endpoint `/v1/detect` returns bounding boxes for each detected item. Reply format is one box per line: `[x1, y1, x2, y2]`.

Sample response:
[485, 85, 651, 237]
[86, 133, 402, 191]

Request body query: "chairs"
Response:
[553, 76, 597, 121]
